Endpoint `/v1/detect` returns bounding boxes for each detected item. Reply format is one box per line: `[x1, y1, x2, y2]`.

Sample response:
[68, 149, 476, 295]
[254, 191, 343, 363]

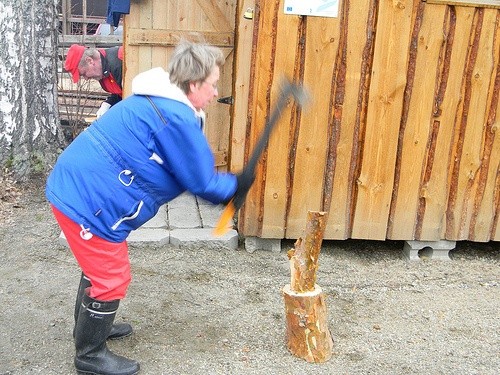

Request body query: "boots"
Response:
[73, 271, 133, 340]
[74, 287, 140, 375]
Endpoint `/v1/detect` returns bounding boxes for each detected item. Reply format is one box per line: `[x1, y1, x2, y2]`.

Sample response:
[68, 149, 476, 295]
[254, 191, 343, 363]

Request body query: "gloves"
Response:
[97, 102, 111, 119]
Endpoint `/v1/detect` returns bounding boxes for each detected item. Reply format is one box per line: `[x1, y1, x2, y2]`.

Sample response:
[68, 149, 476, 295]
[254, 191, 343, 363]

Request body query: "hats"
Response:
[65, 44, 85, 83]
